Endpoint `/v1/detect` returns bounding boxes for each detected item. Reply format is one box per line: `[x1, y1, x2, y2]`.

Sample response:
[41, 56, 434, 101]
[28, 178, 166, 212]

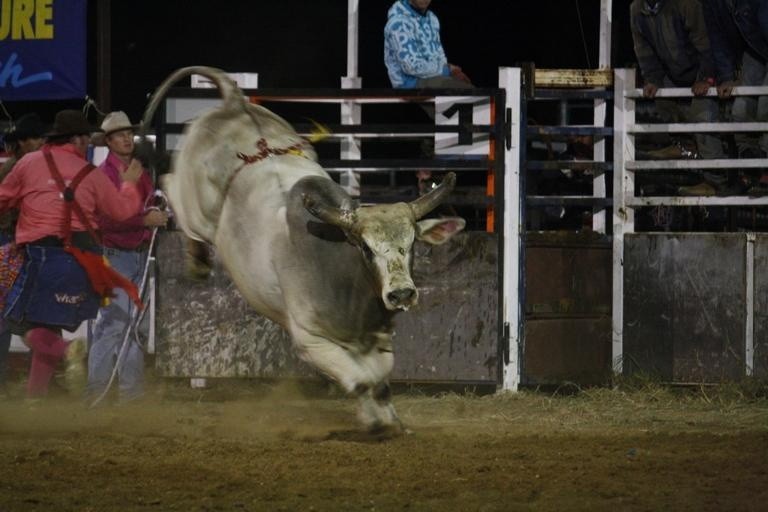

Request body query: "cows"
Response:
[134, 64, 470, 445]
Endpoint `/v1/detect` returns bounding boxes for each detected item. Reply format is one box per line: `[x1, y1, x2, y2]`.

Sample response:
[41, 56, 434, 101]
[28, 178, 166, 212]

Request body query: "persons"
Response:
[2, 112, 49, 157]
[77, 109, 172, 411]
[0, 125, 24, 184]
[548, 115, 617, 234]
[705, 0, 768, 198]
[400, 168, 459, 221]
[0, 109, 149, 404]
[625, 0, 744, 199]
[380, 0, 483, 171]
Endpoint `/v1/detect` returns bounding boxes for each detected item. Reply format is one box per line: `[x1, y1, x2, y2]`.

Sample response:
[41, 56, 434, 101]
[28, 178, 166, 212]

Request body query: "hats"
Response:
[91, 111, 142, 146]
[2, 112, 52, 143]
[45, 109, 105, 137]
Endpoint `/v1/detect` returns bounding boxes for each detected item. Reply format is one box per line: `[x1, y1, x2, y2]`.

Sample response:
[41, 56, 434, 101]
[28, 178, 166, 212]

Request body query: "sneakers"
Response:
[54, 339, 88, 397]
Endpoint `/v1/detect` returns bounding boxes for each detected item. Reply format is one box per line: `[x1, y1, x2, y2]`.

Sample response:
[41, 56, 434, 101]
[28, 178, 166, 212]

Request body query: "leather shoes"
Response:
[677, 176, 768, 196]
[649, 144, 683, 158]
